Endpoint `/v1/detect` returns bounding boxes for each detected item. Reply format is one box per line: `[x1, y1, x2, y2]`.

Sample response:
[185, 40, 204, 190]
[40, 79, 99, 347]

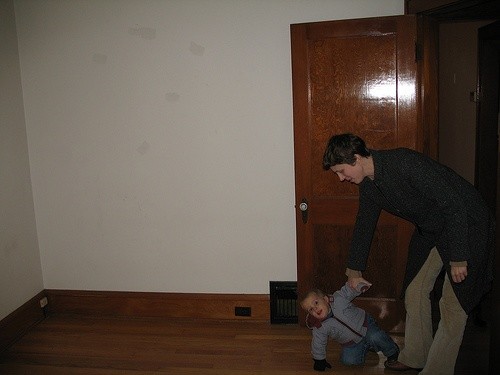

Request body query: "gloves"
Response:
[312, 358, 332, 372]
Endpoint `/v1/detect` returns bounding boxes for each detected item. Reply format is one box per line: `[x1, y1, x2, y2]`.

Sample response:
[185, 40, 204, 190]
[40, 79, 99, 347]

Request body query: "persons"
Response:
[299, 277, 400, 371]
[323, 133, 497, 375]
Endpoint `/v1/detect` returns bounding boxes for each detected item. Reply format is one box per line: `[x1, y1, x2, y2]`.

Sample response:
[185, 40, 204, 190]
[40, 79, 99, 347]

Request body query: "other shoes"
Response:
[388, 361, 423, 372]
[384, 351, 400, 367]
